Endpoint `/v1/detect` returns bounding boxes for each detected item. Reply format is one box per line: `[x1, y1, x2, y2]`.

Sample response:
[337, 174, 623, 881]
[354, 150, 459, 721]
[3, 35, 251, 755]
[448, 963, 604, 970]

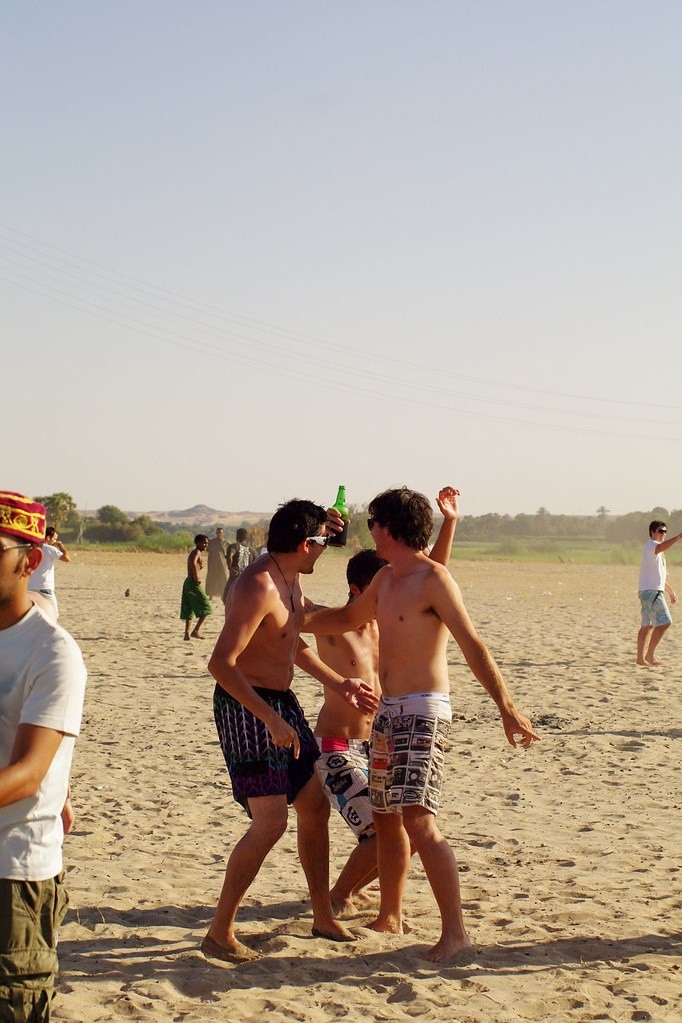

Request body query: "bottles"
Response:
[327, 485, 349, 547]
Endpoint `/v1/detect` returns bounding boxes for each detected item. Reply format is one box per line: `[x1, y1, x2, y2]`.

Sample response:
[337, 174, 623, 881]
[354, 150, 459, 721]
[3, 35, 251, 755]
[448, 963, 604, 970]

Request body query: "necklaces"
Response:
[269, 552, 297, 613]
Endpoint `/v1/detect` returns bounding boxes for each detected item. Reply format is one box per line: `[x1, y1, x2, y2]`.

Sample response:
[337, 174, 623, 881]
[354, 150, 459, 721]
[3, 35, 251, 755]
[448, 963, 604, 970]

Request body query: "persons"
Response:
[293, 484, 461, 918]
[0, 488, 88, 1023]
[180, 534, 213, 640]
[200, 498, 380, 964]
[300, 485, 541, 962]
[206, 528, 270, 604]
[636, 520, 682, 666]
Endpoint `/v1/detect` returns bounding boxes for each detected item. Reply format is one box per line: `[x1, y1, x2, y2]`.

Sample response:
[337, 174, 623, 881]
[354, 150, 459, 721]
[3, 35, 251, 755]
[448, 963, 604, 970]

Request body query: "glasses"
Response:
[367, 519, 376, 530]
[306, 534, 329, 546]
[656, 529, 667, 534]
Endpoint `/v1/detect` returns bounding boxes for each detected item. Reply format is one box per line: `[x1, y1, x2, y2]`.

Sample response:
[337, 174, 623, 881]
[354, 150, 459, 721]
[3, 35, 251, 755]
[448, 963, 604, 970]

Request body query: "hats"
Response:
[0, 491, 47, 543]
